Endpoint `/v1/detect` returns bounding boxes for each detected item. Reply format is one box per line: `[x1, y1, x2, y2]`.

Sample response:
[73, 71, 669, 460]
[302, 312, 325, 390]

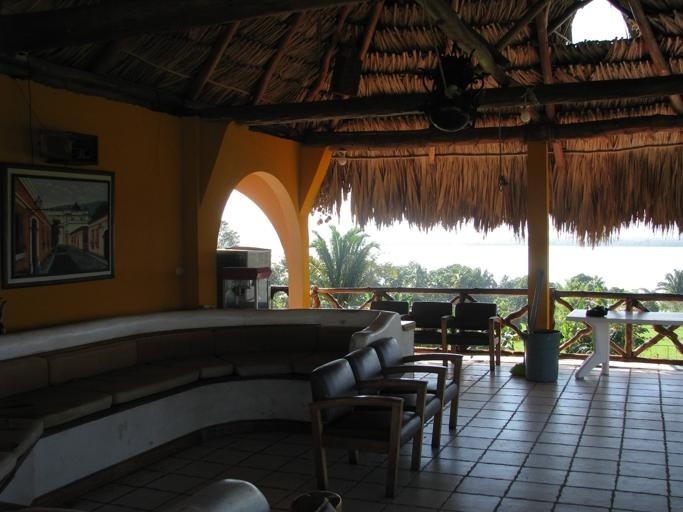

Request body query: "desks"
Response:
[564, 308, 683, 379]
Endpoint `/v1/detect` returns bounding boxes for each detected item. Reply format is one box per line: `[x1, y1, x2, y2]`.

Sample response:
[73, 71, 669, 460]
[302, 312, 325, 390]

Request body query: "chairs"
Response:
[369, 301, 503, 372]
[306, 338, 464, 500]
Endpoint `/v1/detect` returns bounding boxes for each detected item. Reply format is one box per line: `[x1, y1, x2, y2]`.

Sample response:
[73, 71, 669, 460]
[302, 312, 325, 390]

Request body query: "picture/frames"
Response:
[1, 164, 116, 290]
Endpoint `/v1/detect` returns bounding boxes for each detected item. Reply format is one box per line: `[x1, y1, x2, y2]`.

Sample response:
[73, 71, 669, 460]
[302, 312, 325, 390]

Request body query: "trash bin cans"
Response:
[520, 329, 564, 382]
[290, 490, 342, 512]
